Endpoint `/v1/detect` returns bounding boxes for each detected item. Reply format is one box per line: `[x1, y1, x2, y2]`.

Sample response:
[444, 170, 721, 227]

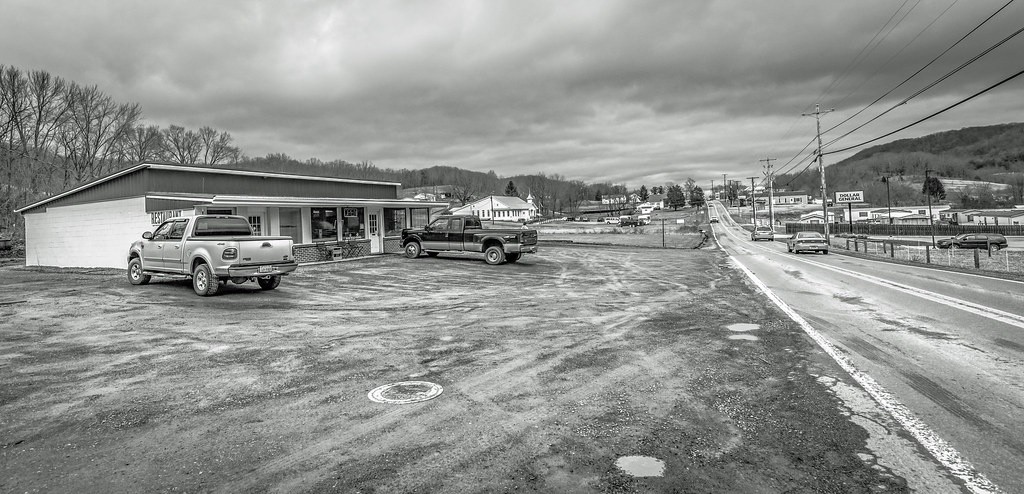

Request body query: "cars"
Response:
[786, 231, 828, 254]
[750, 225, 774, 242]
[562, 216, 645, 228]
[709, 216, 719, 223]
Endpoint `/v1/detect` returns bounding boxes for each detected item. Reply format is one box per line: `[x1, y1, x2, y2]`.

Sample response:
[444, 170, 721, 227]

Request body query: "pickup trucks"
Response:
[398, 214, 540, 265]
[126, 214, 300, 297]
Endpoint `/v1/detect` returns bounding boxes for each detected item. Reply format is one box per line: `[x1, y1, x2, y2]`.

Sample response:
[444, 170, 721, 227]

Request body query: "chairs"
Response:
[348, 239, 364, 257]
[337, 240, 352, 259]
[316, 243, 332, 262]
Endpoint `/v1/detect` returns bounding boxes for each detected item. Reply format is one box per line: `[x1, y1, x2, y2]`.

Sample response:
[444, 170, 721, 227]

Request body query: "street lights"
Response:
[881, 176, 891, 237]
[924, 167, 937, 250]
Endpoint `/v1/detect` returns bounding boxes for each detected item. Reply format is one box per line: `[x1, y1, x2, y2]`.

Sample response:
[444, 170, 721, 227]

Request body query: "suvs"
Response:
[936, 232, 1008, 251]
